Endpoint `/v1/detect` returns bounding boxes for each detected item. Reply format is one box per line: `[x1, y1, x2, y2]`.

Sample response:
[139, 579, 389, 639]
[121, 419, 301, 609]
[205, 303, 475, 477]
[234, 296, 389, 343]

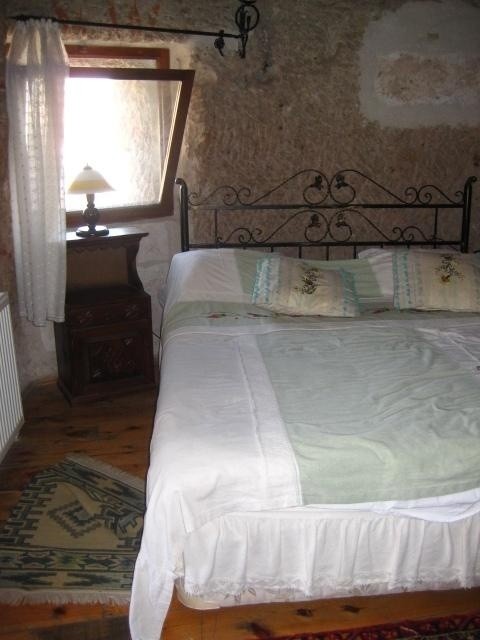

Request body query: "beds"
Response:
[142, 159, 479, 610]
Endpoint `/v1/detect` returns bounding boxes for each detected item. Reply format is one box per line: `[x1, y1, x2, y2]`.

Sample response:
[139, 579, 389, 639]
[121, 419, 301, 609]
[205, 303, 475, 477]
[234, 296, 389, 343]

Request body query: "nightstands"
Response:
[53, 225, 156, 407]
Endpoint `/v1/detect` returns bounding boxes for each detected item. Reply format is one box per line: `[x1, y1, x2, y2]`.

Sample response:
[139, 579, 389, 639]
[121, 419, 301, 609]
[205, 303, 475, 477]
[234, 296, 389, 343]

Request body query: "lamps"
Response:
[66, 162, 115, 237]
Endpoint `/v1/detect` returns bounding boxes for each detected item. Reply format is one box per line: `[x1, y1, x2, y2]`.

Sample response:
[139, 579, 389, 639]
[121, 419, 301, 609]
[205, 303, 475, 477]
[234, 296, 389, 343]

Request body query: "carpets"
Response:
[0, 450, 150, 607]
[253, 611, 479, 639]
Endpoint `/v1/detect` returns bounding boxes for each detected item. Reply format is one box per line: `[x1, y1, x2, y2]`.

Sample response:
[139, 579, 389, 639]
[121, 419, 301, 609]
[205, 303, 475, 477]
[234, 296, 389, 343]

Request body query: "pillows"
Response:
[169, 249, 273, 319]
[254, 257, 363, 318]
[358, 250, 396, 296]
[392, 246, 480, 313]
